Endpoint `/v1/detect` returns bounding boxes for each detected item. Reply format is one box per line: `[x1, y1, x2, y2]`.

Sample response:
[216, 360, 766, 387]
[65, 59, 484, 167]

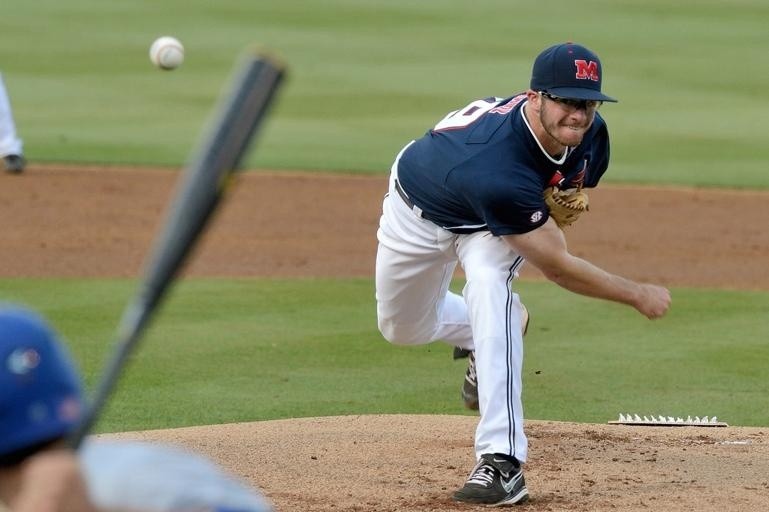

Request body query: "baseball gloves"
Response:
[543, 186, 588, 227]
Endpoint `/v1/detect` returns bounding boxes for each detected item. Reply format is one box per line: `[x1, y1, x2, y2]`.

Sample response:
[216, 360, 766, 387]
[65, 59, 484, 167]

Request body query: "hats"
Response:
[529, 38, 619, 104]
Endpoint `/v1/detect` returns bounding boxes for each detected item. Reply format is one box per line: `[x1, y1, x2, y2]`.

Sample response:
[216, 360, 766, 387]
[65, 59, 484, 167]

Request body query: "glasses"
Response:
[536, 89, 604, 111]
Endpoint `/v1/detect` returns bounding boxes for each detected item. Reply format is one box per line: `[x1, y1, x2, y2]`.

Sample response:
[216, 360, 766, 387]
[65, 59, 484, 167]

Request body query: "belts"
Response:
[393, 168, 460, 228]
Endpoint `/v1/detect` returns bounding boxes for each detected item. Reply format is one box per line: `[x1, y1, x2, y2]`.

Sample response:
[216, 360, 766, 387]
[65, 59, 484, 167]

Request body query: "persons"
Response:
[0, 301, 277, 512]
[0, 67, 25, 173]
[375, 41, 673, 505]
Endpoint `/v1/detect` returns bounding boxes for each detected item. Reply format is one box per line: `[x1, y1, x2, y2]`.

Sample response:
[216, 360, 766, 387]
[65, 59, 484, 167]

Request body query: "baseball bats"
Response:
[70, 51, 285, 453]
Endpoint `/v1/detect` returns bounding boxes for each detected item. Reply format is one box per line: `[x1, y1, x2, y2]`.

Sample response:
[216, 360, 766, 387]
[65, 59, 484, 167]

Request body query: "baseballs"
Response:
[150, 37, 184, 71]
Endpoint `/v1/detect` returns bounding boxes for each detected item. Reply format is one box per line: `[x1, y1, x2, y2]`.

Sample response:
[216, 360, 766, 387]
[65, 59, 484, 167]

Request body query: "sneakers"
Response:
[451, 305, 532, 413]
[452, 450, 531, 509]
[3, 154, 26, 177]
